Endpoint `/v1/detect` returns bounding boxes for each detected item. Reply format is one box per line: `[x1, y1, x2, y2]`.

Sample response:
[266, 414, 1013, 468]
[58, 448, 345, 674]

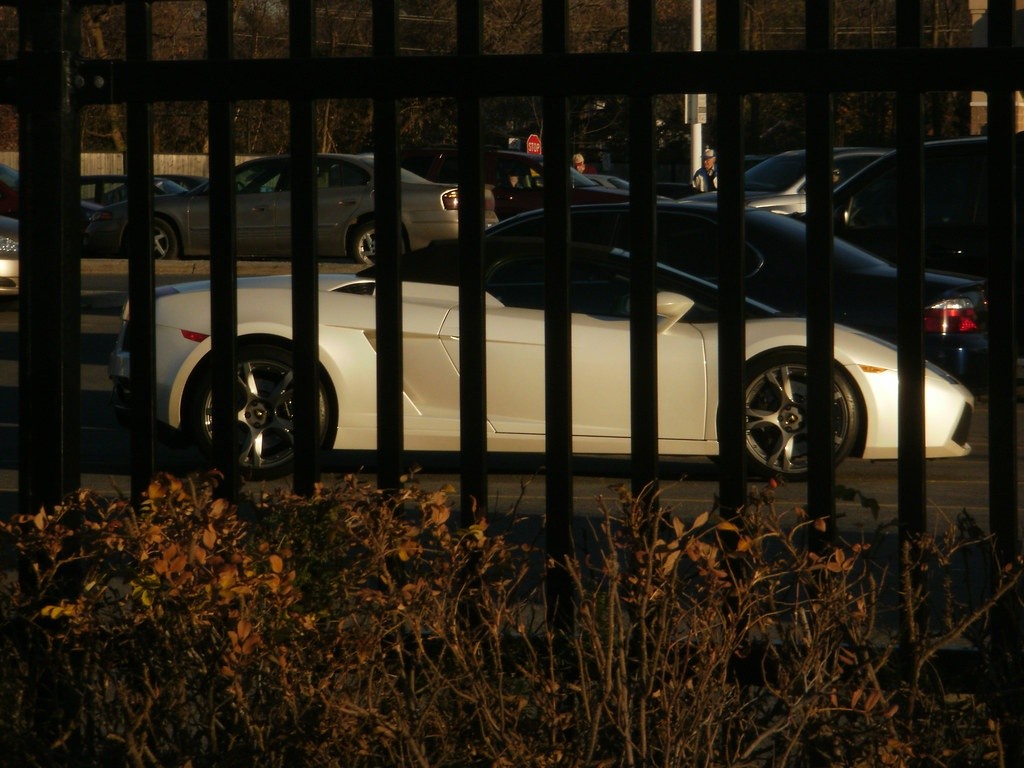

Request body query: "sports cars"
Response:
[112, 240, 979, 503]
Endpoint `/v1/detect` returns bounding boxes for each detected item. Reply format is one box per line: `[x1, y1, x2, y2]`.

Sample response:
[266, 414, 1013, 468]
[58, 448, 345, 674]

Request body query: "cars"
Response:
[84, 173, 210, 205]
[679, 147, 896, 215]
[0, 161, 104, 228]
[0, 216, 20, 294]
[83, 152, 500, 266]
[583, 172, 674, 201]
[793, 131, 1024, 280]
[354, 196, 991, 395]
[418, 144, 674, 221]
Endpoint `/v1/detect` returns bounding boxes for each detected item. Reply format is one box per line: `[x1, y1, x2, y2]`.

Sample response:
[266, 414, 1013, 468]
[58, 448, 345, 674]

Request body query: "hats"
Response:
[574, 154, 584, 163]
[701, 149, 714, 158]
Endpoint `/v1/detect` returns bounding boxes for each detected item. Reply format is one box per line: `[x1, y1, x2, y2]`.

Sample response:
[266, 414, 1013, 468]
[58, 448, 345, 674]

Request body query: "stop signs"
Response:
[527, 135, 542, 154]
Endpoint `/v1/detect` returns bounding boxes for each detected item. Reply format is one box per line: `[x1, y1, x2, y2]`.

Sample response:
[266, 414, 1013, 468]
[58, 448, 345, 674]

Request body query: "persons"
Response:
[573, 154, 597, 174]
[692, 149, 718, 192]
[833, 166, 842, 190]
